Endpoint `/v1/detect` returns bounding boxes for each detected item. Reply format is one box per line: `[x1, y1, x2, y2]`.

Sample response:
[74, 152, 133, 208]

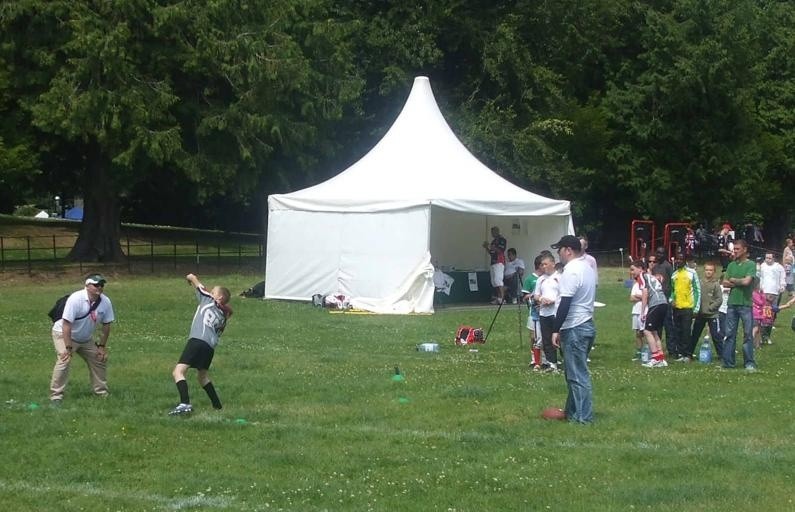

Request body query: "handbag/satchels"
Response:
[48, 295, 69, 322]
[455, 327, 483, 345]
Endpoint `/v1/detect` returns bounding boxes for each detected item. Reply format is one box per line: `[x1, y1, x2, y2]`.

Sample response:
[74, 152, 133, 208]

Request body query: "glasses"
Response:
[92, 283, 104, 287]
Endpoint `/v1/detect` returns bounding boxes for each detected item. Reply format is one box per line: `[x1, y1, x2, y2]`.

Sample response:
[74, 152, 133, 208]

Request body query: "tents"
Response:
[34, 210, 48, 218]
[56, 205, 83, 220]
[259, 73, 577, 316]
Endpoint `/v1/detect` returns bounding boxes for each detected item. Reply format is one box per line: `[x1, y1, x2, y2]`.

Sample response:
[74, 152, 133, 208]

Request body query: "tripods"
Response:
[482, 267, 531, 351]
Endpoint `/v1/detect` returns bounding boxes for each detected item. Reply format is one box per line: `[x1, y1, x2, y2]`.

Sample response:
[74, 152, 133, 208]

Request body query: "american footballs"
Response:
[219, 305, 233, 316]
[542, 408, 566, 420]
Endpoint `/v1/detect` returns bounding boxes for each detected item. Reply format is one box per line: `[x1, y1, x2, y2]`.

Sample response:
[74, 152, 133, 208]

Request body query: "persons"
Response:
[503, 246, 524, 304]
[168, 274, 233, 416]
[47, 271, 115, 402]
[628, 221, 795, 373]
[482, 226, 507, 305]
[520, 231, 598, 373]
[549, 235, 598, 424]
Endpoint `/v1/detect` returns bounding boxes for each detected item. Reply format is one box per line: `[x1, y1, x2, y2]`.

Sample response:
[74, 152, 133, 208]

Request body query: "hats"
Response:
[85, 274, 106, 286]
[723, 225, 732, 231]
[551, 235, 581, 250]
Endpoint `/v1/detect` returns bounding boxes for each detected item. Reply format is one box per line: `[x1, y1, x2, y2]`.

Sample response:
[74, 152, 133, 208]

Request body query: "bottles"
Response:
[700, 334, 712, 364]
[415, 342, 441, 353]
[642, 346, 650, 365]
[529, 298, 539, 321]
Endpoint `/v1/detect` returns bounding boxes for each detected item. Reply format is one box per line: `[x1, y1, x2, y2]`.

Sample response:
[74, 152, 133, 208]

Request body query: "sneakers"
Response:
[490, 298, 518, 305]
[168, 404, 193, 415]
[529, 360, 561, 374]
[632, 352, 693, 368]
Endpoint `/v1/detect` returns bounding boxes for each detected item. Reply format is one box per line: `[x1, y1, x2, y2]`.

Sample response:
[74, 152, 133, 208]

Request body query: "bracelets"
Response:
[65, 345, 71, 349]
[94, 341, 104, 348]
